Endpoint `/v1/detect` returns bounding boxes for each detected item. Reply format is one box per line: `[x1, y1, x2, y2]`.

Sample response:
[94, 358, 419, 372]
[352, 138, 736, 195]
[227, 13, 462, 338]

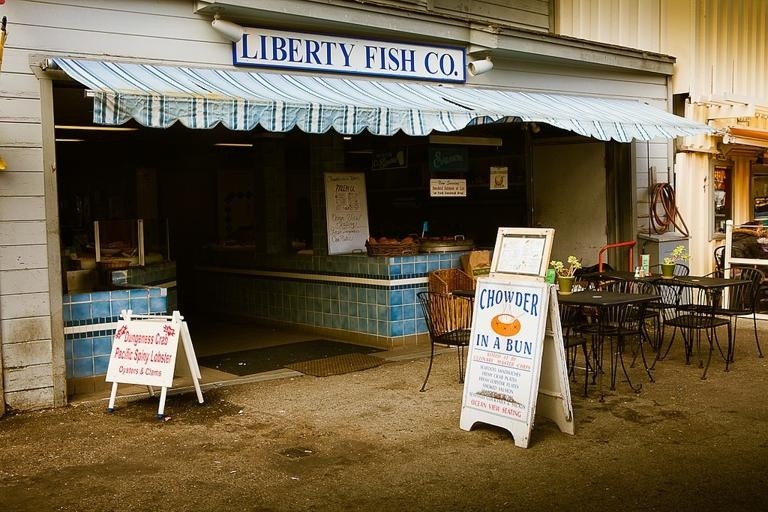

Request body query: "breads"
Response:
[368, 237, 413, 244]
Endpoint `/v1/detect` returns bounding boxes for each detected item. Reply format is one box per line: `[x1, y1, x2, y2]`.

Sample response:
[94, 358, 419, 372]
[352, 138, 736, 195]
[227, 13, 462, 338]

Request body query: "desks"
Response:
[654, 276, 753, 358]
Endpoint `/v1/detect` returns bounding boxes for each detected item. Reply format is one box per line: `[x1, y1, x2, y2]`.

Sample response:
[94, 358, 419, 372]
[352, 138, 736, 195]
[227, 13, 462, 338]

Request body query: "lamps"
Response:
[466, 55, 494, 78]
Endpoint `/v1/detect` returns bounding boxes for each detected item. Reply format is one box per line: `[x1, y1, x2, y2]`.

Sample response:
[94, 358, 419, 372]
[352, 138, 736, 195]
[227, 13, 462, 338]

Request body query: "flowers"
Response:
[663, 244, 691, 265]
[550, 255, 583, 277]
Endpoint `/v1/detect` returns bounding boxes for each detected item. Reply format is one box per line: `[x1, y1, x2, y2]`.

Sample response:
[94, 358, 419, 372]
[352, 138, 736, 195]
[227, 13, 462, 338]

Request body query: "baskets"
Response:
[365, 239, 422, 256]
[98, 261, 131, 269]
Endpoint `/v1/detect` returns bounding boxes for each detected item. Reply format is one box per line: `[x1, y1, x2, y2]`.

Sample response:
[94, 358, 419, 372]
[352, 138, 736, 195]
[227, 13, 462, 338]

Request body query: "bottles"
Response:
[634, 267, 644, 277]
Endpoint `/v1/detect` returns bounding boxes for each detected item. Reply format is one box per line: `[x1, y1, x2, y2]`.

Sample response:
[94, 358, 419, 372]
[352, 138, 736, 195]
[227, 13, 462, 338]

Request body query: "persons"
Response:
[719, 221, 768, 314]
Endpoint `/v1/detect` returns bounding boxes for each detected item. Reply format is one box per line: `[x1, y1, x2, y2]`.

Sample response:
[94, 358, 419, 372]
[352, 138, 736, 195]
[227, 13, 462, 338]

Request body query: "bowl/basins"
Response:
[100, 249, 122, 256]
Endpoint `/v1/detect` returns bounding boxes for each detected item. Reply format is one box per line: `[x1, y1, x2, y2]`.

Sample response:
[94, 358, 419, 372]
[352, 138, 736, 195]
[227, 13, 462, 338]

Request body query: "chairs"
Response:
[652, 277, 731, 380]
[555, 261, 689, 400]
[416, 290, 477, 393]
[704, 267, 767, 364]
